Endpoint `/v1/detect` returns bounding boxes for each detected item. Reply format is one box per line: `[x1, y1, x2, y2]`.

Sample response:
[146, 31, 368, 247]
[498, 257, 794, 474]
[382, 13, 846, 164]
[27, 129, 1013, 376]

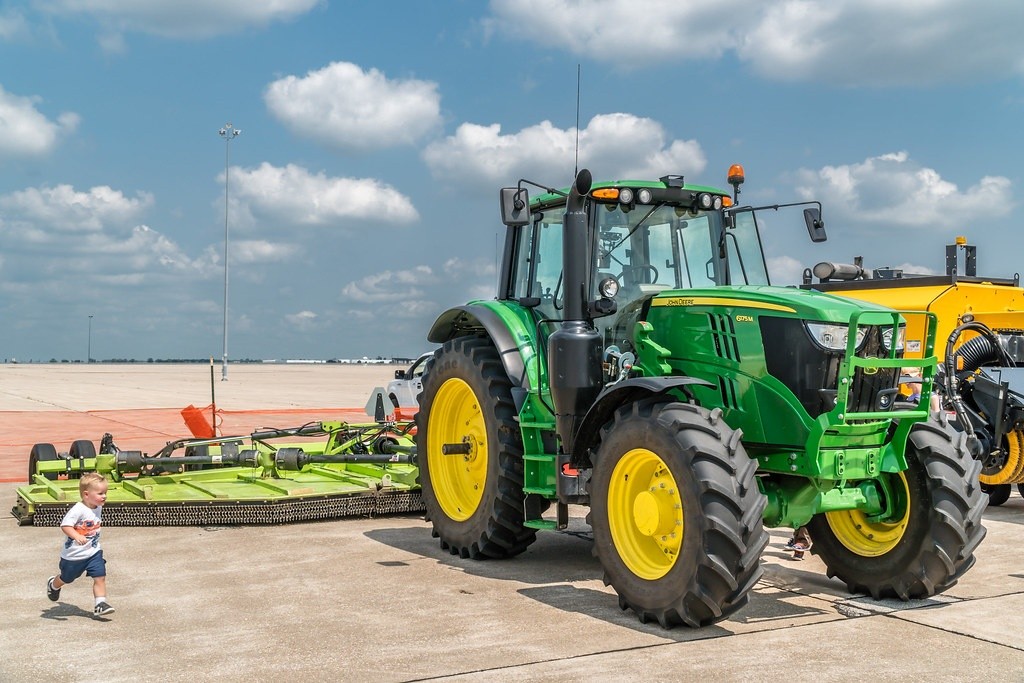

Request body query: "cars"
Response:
[387, 352, 434, 421]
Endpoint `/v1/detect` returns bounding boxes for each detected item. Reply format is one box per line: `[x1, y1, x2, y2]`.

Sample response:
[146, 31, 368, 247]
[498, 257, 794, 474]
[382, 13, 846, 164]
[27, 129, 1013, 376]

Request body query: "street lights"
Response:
[88, 315, 93, 362]
[219, 122, 241, 381]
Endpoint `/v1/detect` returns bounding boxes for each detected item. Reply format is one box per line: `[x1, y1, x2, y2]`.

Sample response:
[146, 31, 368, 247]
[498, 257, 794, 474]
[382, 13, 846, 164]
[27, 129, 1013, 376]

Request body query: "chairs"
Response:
[596, 273, 620, 295]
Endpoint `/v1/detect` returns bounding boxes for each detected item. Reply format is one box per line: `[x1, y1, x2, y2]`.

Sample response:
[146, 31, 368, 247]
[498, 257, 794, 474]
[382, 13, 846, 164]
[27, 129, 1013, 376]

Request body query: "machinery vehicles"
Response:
[785, 237, 1024, 505]
[413, 164, 991, 631]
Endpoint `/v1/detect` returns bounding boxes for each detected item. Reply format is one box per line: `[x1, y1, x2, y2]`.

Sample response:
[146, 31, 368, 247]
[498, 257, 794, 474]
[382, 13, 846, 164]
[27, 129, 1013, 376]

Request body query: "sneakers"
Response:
[94, 602, 115, 618]
[47, 576, 60, 602]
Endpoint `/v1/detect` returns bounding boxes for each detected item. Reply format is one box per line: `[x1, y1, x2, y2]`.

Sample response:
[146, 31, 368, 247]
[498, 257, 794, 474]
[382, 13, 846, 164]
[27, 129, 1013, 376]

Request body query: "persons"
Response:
[46, 472, 115, 616]
